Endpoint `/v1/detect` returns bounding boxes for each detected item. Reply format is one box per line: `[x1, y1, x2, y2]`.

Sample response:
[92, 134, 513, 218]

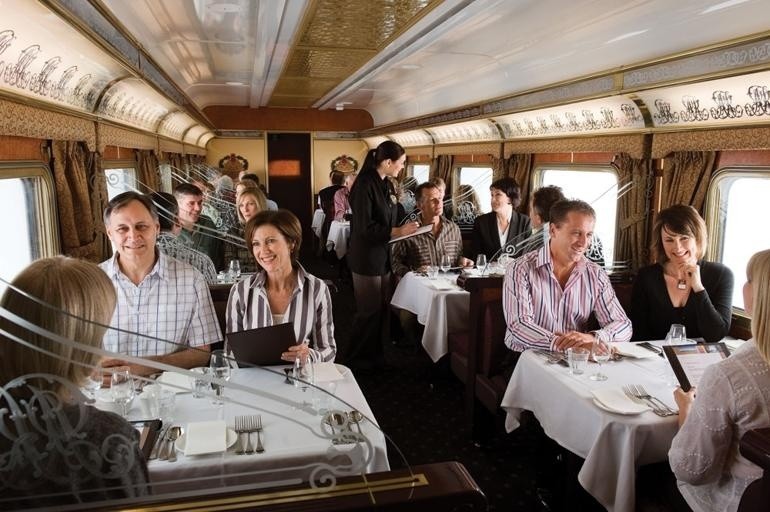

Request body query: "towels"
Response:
[614, 340, 652, 359]
[160, 370, 192, 396]
[306, 359, 345, 381]
[183, 419, 227, 457]
[591, 387, 654, 416]
[431, 279, 452, 292]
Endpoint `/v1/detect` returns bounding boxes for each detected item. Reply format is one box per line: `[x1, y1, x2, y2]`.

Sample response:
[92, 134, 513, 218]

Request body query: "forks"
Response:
[234, 415, 265, 456]
[626, 383, 679, 417]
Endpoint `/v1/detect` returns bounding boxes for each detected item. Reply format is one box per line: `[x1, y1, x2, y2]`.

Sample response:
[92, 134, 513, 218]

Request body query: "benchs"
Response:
[203, 285, 232, 352]
[451, 270, 637, 435]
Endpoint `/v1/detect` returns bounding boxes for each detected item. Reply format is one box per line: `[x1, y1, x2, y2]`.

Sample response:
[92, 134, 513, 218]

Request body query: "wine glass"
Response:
[664, 323, 687, 385]
[476, 254, 487, 276]
[84, 350, 315, 425]
[440, 255, 451, 275]
[590, 332, 612, 382]
[229, 260, 241, 278]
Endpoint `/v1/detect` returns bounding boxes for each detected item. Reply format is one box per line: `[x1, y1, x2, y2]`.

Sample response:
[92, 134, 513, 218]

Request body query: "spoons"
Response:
[167, 427, 184, 462]
[325, 411, 364, 444]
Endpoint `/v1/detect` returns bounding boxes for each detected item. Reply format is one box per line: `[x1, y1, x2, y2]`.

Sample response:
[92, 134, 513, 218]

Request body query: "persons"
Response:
[464, 177, 531, 269]
[144, 167, 280, 286]
[390, 182, 464, 396]
[646, 248, 769, 512]
[348, 140, 408, 382]
[501, 200, 633, 511]
[317, 169, 482, 285]
[628, 202, 733, 343]
[502, 184, 605, 274]
[88, 191, 225, 389]
[220, 207, 338, 368]
[2, 255, 154, 512]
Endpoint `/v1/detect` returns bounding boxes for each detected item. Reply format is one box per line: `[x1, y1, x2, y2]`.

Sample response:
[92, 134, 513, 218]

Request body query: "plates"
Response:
[592, 397, 649, 415]
[174, 428, 237, 454]
[660, 338, 698, 349]
[422, 277, 455, 290]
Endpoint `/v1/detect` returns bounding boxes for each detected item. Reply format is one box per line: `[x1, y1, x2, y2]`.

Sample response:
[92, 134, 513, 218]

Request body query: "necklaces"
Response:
[669, 260, 692, 290]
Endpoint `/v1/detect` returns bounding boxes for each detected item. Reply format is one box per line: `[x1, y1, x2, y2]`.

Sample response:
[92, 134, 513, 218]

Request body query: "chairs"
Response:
[93, 460, 487, 511]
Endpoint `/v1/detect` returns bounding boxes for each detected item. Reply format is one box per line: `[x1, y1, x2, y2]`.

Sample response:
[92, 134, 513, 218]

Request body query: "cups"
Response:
[219, 270, 232, 282]
[312, 380, 338, 417]
[427, 266, 439, 280]
[567, 347, 591, 375]
